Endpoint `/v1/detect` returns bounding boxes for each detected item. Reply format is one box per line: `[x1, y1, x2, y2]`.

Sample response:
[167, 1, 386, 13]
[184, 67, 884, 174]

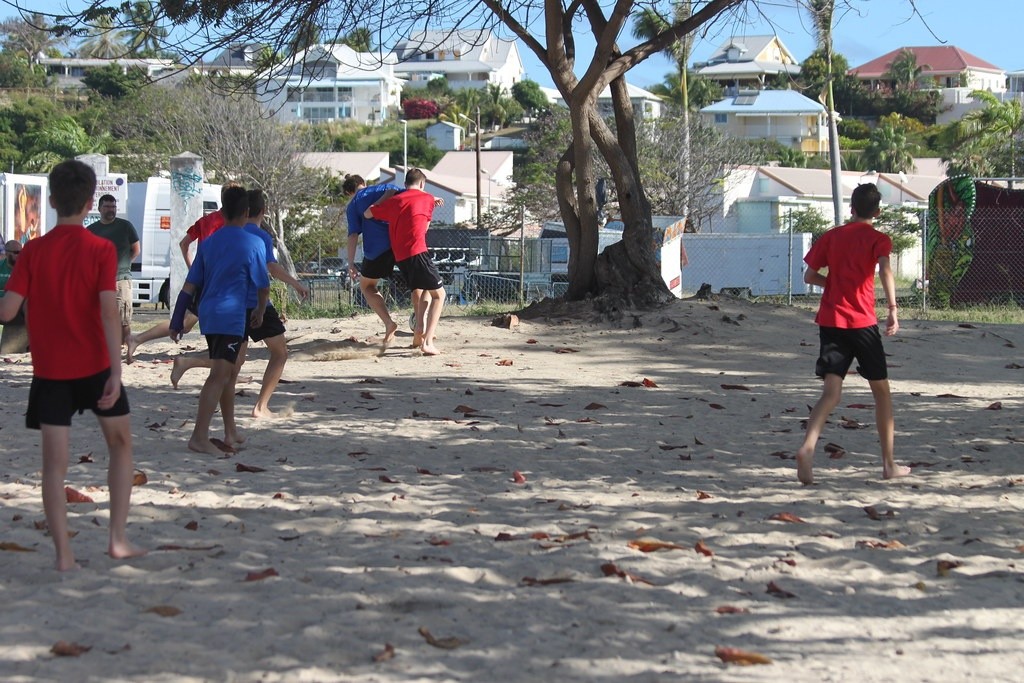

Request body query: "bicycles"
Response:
[440, 266, 483, 310]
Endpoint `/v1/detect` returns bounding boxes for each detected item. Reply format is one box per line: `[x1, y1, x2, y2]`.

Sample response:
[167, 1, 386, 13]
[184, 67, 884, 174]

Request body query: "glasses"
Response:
[101, 205, 117, 209]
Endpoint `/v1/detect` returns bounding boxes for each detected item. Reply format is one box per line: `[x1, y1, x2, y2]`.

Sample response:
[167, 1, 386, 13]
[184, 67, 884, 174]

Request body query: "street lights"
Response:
[478, 167, 507, 224]
[398, 117, 410, 192]
[459, 105, 484, 229]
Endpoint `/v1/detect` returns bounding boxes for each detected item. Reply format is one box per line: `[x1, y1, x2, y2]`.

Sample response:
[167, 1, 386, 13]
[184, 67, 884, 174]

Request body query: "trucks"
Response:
[123, 175, 234, 310]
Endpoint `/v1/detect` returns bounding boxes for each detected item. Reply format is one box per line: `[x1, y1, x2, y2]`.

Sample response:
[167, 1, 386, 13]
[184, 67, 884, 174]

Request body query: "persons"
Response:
[169, 185, 272, 456]
[363, 168, 447, 358]
[126, 206, 230, 366]
[343, 174, 444, 343]
[0, 161, 147, 575]
[794, 183, 912, 484]
[169, 190, 313, 419]
[84, 192, 143, 349]
[0, 241, 27, 302]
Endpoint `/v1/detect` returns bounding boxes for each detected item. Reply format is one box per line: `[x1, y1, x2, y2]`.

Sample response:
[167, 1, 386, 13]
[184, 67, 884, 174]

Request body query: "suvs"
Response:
[316, 257, 347, 282]
[341, 261, 367, 290]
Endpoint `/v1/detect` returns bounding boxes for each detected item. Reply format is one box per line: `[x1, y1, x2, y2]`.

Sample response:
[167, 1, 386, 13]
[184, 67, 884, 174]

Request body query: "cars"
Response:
[297, 261, 319, 282]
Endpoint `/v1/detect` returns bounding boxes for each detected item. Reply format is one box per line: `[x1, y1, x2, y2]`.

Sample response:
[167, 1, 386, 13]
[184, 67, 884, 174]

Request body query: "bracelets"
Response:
[888, 304, 897, 309]
